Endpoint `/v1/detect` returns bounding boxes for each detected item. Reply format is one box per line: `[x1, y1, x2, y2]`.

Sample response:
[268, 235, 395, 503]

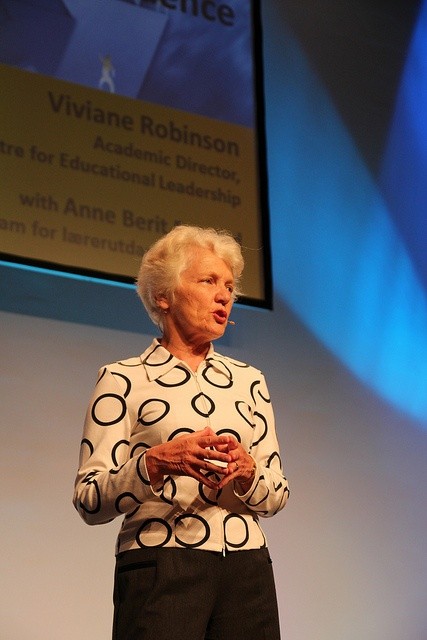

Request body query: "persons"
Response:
[71, 226, 291, 640]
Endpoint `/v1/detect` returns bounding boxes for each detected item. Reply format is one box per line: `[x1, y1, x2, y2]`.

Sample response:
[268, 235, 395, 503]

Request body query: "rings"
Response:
[234, 461, 239, 470]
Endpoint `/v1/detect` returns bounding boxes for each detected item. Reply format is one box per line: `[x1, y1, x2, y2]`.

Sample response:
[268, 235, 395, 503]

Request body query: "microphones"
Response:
[227, 321, 235, 325]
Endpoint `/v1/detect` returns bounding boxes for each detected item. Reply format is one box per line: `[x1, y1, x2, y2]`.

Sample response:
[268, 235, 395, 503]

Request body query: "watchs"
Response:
[240, 457, 257, 485]
